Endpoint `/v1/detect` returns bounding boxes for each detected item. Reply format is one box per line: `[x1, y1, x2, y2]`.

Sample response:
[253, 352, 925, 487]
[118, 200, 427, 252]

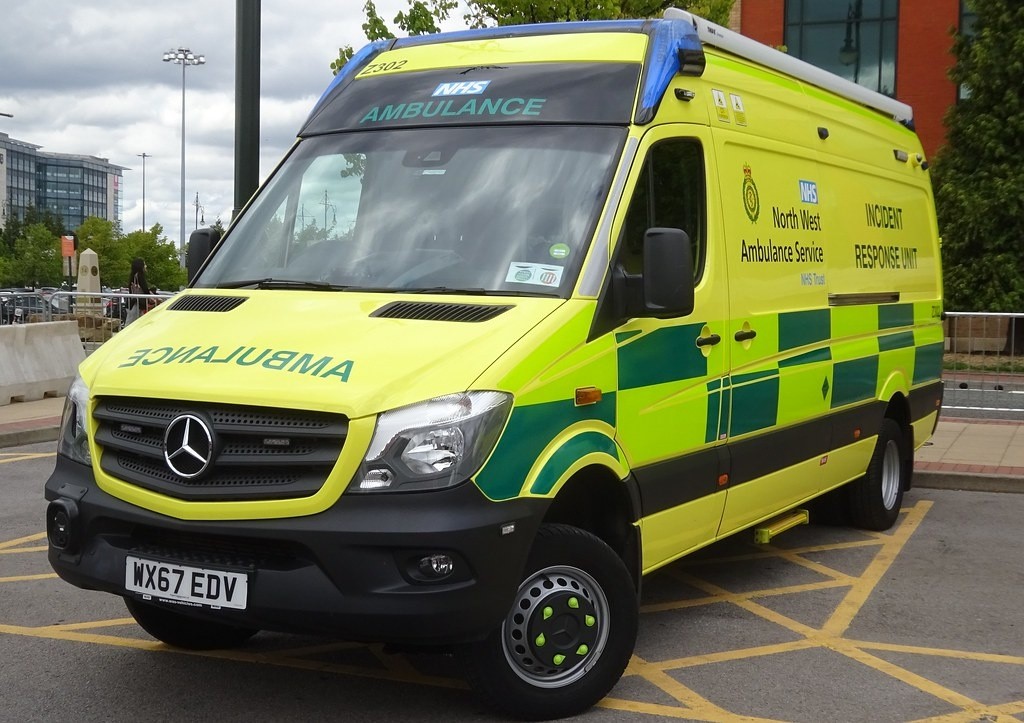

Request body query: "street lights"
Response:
[135, 152, 152, 235]
[162, 46, 208, 293]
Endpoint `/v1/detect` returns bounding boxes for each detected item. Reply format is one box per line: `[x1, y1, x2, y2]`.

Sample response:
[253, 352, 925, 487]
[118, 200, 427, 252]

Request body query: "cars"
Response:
[0, 285, 128, 319]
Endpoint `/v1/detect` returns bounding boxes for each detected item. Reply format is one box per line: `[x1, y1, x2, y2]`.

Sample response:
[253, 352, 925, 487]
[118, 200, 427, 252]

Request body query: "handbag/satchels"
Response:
[130, 273, 144, 294]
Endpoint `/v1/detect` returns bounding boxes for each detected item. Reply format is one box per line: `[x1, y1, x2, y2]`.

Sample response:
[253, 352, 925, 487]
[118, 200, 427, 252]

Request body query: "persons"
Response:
[125, 259, 150, 327]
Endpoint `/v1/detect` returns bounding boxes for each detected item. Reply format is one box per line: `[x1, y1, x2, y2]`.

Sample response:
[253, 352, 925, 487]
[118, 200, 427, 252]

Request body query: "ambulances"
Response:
[40, 6, 947, 719]
